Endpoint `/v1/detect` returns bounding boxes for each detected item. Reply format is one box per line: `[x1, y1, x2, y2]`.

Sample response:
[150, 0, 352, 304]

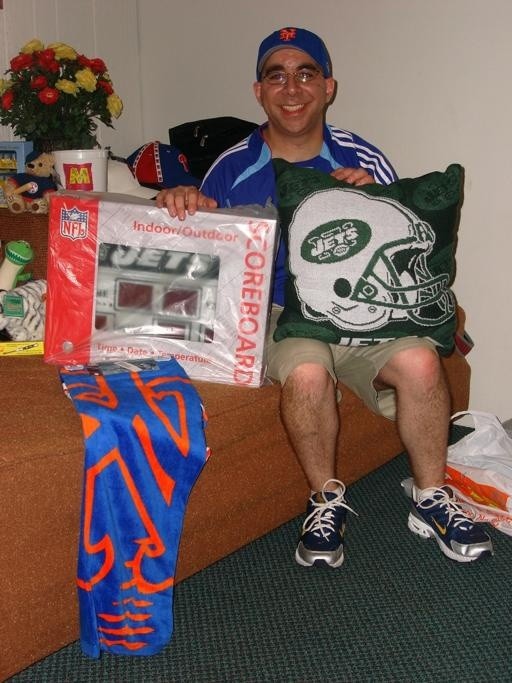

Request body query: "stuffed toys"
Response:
[0, 239, 47, 342]
[5, 150, 57, 214]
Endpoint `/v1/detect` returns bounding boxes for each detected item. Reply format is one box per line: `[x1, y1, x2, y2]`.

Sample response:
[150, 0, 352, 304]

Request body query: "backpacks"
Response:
[169, 117, 260, 180]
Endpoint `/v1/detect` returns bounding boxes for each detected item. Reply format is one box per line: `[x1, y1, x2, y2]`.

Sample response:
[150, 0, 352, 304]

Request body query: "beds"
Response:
[1, 199, 472, 680]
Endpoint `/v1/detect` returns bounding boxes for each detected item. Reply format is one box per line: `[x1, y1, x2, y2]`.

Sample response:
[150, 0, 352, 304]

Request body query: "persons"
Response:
[155, 28, 494, 569]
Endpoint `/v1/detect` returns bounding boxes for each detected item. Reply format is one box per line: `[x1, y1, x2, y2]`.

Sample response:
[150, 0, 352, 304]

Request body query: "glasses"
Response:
[261, 67, 320, 85]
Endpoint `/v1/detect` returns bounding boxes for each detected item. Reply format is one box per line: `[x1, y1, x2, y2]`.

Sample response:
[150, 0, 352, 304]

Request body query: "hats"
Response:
[257, 27, 332, 82]
[127, 141, 202, 189]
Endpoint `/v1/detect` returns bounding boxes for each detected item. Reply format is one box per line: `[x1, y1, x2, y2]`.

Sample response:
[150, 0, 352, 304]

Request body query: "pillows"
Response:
[272, 163, 465, 356]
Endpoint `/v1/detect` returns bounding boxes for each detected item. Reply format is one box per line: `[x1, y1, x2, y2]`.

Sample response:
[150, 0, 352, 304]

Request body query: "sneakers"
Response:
[295, 479, 359, 569]
[406, 485, 495, 564]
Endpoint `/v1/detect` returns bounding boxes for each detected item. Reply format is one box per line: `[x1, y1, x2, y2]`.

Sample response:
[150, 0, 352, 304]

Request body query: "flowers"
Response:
[0, 37, 124, 150]
